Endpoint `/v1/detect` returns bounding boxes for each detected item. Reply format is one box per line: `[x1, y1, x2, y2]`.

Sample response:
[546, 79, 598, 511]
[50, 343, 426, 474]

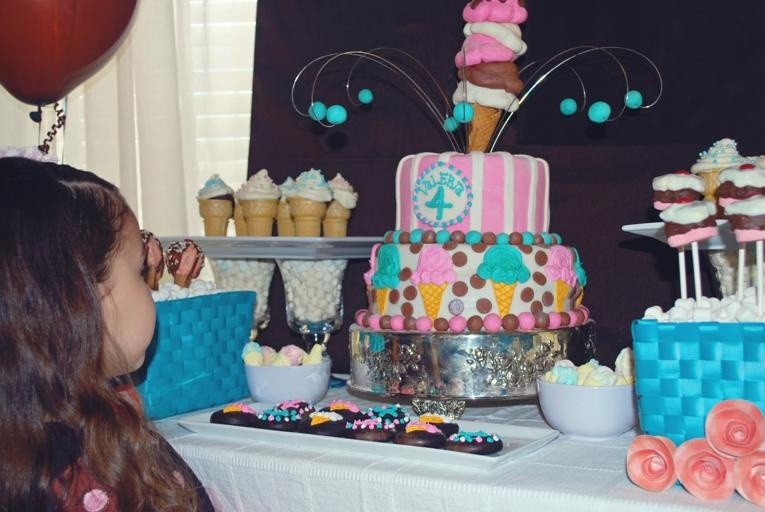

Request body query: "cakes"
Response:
[356, 1, 589, 333]
[210, 400, 503, 455]
[651, 163, 765, 248]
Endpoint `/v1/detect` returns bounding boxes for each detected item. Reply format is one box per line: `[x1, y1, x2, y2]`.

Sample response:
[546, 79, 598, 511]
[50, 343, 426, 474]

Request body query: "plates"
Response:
[157, 237, 384, 260]
[179, 401, 560, 472]
[617, 214, 742, 252]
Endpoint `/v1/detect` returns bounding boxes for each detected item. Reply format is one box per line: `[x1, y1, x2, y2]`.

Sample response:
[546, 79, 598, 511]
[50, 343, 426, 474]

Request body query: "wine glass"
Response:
[276, 260, 349, 389]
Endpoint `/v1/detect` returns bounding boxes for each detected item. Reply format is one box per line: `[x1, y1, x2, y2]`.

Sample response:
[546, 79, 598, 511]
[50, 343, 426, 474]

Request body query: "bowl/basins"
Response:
[537, 375, 639, 442]
[244, 357, 332, 403]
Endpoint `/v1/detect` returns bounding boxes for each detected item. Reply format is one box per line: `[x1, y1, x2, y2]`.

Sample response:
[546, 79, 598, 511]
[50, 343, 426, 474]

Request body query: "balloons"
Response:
[0, 1, 138, 124]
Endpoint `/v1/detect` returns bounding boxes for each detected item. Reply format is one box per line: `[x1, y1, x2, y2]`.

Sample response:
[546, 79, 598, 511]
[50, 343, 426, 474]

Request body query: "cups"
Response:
[209, 258, 276, 340]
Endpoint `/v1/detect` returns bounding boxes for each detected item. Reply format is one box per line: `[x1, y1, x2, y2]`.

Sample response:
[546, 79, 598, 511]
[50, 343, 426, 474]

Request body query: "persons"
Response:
[0, 154, 218, 512]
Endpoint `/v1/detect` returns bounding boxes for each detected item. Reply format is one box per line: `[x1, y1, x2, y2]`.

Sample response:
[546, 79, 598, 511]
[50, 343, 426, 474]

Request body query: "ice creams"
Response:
[691, 138, 765, 221]
[139, 169, 359, 291]
